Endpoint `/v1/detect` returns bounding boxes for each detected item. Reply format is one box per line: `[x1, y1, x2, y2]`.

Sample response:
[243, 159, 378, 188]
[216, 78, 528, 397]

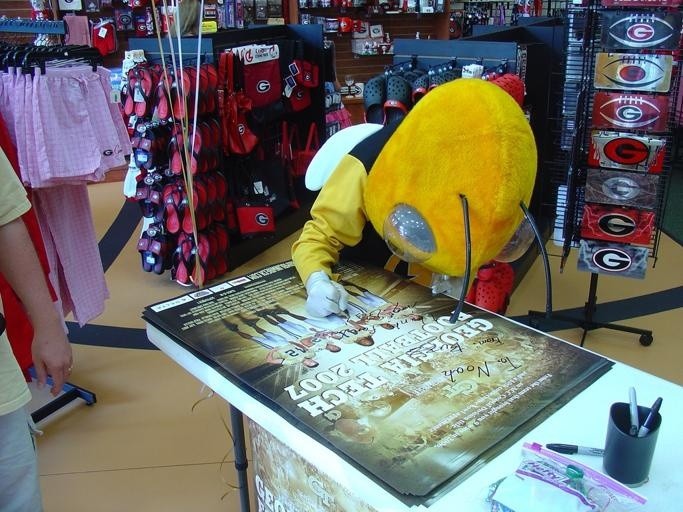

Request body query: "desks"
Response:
[145, 253, 683, 512]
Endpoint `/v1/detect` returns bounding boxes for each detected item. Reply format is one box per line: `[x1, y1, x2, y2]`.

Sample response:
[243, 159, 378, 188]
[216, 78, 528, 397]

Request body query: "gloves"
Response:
[304, 270, 349, 317]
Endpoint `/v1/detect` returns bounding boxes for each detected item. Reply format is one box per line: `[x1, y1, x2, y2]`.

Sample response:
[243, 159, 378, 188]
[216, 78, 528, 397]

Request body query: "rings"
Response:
[65, 366, 72, 373]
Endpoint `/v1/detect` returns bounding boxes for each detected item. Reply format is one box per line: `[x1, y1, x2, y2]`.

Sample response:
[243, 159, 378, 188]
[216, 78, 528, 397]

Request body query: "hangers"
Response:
[0, 18, 100, 75]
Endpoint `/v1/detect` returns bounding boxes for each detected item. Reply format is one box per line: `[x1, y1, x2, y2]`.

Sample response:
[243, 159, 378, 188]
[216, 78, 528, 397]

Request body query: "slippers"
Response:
[475, 259, 515, 314]
[363, 66, 524, 123]
[120, 63, 228, 287]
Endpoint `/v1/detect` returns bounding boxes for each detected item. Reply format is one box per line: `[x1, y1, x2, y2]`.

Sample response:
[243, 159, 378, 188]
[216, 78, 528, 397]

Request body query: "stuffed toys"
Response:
[287, 75, 554, 330]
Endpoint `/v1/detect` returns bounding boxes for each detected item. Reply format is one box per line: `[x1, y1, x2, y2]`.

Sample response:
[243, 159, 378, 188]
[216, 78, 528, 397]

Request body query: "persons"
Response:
[220, 280, 424, 370]
[1, 149, 74, 512]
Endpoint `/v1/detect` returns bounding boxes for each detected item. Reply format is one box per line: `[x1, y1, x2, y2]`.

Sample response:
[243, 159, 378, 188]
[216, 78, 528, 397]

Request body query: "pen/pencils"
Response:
[545, 443, 604, 457]
[628, 386, 639, 434]
[638, 397, 663, 437]
[320, 269, 350, 319]
